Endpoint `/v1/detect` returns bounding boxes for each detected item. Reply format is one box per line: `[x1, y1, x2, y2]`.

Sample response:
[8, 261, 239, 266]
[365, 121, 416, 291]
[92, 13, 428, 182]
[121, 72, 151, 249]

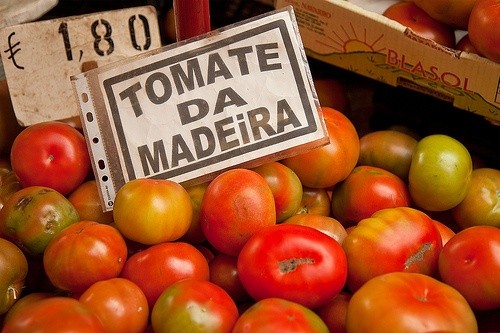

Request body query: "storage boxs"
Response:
[274, 0, 500, 126]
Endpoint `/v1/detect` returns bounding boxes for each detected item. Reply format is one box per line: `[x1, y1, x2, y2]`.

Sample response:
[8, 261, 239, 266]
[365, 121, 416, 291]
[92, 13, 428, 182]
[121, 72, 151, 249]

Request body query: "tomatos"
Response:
[382, 0, 500, 66]
[165, 7, 176, 39]
[0, 70, 500, 333]
